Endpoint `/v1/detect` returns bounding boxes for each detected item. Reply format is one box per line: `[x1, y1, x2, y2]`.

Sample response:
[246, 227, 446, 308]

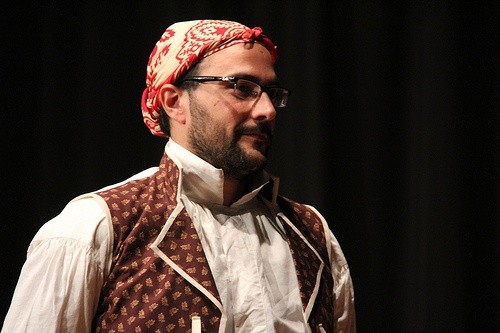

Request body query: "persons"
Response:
[2, 20, 355, 333]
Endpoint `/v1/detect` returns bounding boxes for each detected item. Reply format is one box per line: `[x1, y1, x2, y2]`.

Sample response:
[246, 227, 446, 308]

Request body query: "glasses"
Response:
[180, 75, 290, 109]
